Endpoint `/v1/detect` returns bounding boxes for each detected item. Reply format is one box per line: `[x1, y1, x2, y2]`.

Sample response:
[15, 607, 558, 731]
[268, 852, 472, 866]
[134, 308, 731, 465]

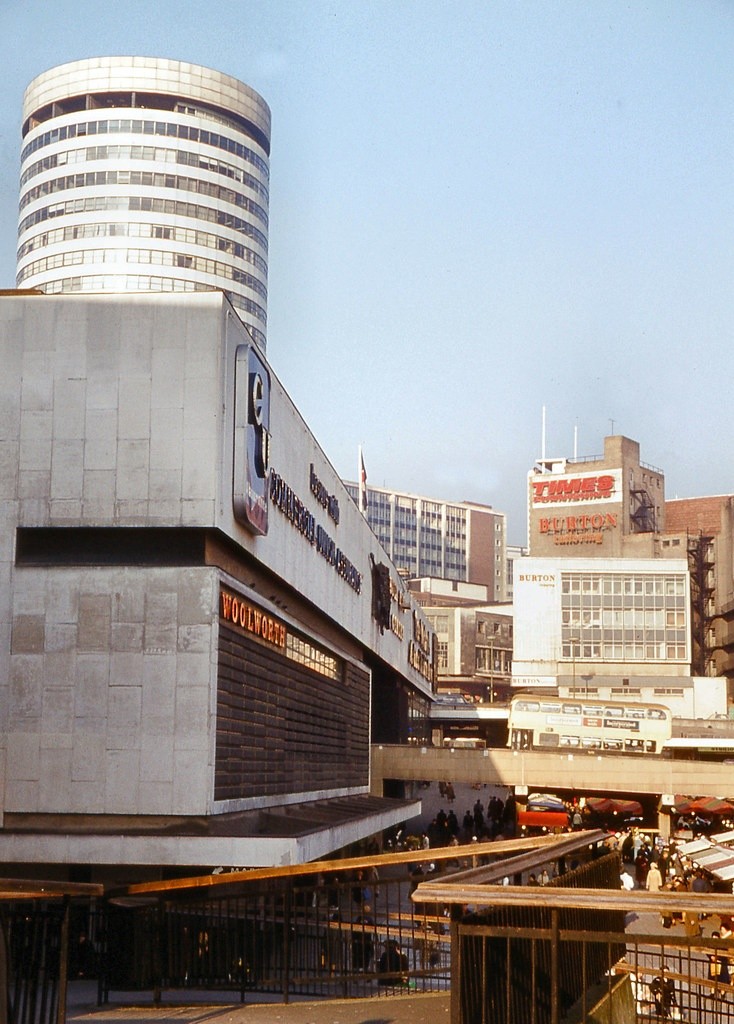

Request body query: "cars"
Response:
[431, 694, 477, 710]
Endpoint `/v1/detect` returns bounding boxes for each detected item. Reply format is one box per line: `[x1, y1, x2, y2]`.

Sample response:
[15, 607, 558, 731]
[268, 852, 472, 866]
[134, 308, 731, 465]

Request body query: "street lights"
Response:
[487, 635, 495, 703]
[569, 637, 579, 698]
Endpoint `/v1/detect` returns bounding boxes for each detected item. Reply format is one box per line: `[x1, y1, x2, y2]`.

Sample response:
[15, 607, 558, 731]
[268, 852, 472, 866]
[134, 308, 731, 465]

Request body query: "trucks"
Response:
[449, 737, 486, 749]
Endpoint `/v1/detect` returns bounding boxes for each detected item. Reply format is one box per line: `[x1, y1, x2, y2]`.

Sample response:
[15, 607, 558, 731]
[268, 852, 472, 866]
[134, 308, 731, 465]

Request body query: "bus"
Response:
[505, 693, 672, 754]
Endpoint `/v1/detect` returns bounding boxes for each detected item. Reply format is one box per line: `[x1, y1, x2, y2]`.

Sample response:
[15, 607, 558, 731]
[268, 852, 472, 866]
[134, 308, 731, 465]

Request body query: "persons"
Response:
[179, 814, 734, 1016]
[419, 781, 515, 828]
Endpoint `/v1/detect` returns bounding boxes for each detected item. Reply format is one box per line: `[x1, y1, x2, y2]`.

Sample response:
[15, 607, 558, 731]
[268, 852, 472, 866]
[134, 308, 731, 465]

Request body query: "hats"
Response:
[651, 863, 657, 868]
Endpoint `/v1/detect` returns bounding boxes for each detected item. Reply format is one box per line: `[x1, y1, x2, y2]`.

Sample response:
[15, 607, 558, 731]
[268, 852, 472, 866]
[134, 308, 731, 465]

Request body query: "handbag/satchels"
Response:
[710, 959, 722, 976]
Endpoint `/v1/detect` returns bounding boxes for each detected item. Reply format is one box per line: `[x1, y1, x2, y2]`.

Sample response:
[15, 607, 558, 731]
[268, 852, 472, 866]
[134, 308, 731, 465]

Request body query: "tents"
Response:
[528, 793, 564, 809]
[674, 795, 734, 813]
[587, 798, 644, 815]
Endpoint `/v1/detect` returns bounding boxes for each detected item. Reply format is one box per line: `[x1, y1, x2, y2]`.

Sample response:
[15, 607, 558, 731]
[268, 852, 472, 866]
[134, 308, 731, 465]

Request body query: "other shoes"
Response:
[721, 993, 725, 1001]
[709, 994, 714, 999]
